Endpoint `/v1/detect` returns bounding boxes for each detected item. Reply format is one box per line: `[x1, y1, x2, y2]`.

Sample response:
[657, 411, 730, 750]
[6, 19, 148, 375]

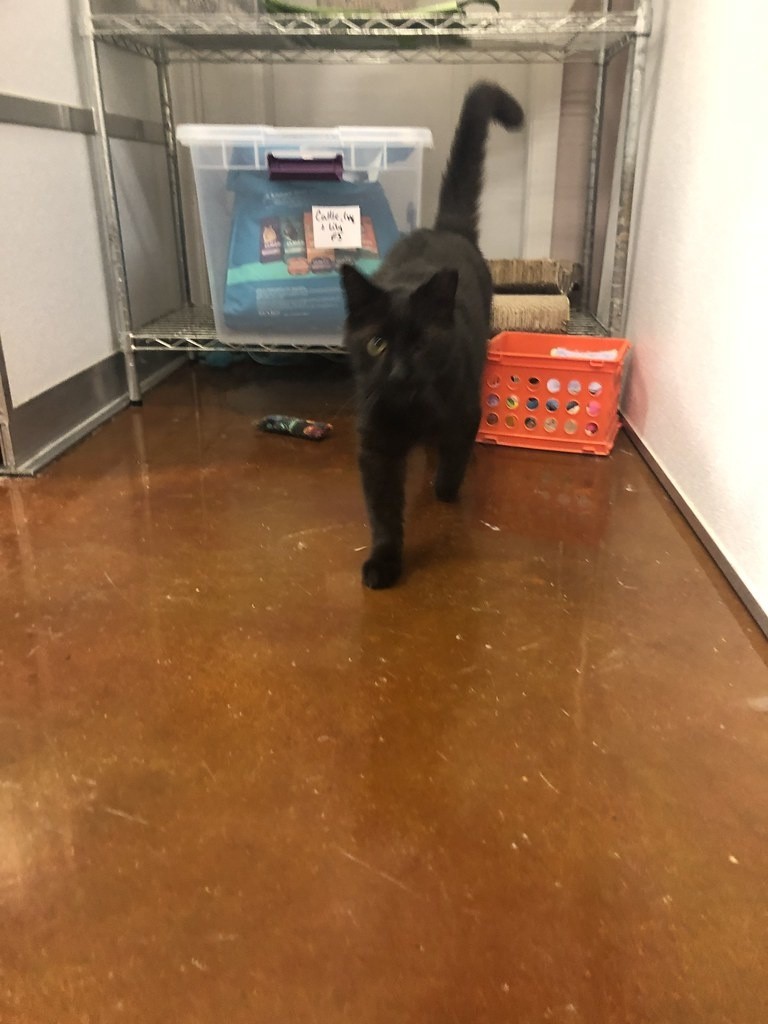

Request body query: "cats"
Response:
[338, 79, 525, 592]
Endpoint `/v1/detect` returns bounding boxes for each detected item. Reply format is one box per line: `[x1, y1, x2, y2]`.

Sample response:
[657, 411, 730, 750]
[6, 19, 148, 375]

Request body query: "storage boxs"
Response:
[473, 330, 630, 457]
[175, 123, 434, 346]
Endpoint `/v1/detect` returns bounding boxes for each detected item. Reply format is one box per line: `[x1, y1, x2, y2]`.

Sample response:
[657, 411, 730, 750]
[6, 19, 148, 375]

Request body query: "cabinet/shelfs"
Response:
[71, 0, 653, 407]
